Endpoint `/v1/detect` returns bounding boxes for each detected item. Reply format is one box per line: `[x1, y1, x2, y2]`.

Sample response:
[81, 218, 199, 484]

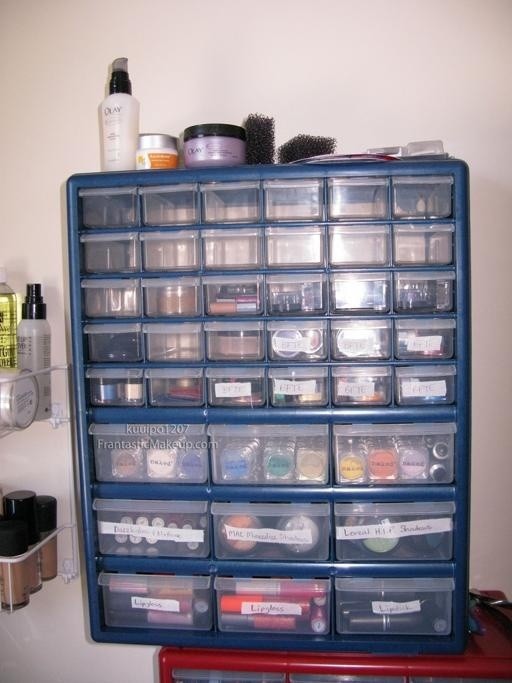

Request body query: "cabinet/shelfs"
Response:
[1, 366, 77, 617]
[151, 586, 512, 681]
[60, 151, 476, 660]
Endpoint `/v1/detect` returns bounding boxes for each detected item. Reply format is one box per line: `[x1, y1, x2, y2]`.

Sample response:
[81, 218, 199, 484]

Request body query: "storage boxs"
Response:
[74, 186, 456, 639]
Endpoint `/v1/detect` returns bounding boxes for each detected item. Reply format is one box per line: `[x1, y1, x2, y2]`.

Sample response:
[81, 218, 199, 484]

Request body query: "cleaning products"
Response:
[100, 57, 139, 171]
[18, 283, 51, 420]
